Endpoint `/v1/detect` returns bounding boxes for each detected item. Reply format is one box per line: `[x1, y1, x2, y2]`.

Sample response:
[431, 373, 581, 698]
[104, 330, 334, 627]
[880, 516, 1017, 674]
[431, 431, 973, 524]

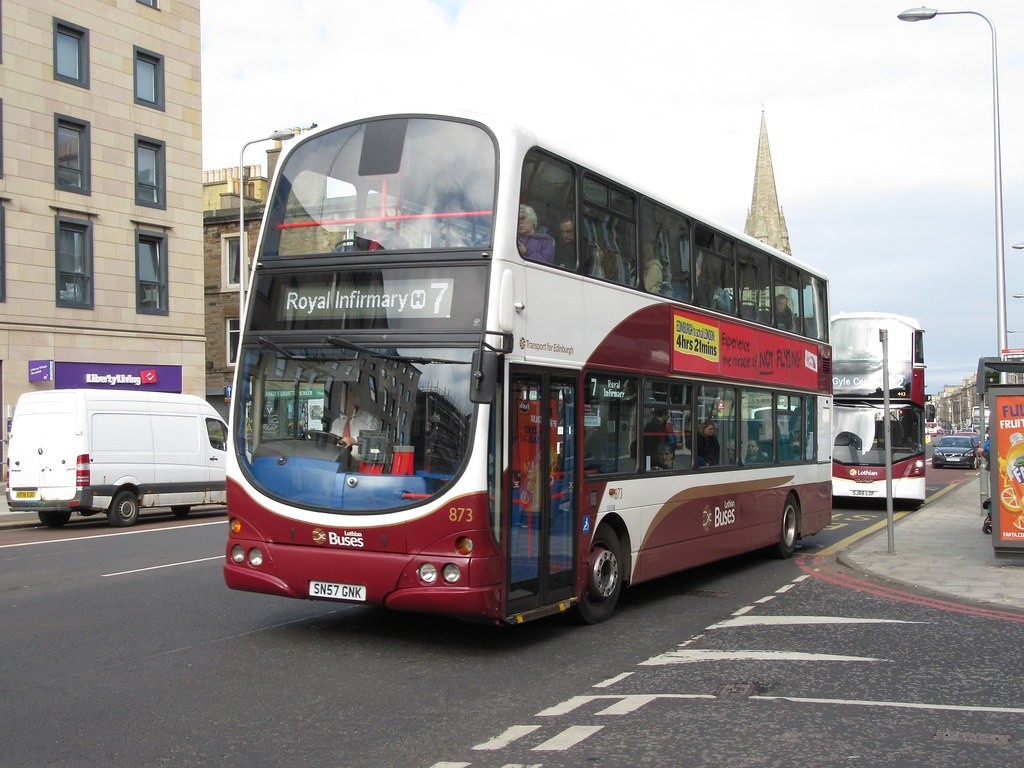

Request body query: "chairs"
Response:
[470, 237, 806, 337]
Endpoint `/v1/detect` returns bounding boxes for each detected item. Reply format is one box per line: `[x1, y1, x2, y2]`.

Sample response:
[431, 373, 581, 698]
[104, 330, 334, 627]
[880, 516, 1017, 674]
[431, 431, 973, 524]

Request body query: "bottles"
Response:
[1005, 432, 1024, 515]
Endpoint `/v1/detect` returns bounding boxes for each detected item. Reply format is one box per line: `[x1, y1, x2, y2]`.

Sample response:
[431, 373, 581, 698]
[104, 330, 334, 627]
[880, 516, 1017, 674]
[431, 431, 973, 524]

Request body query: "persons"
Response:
[787, 415, 810, 460]
[554, 218, 595, 274]
[353, 207, 409, 251]
[644, 243, 663, 294]
[775, 294, 793, 330]
[713, 287, 734, 314]
[904, 435, 916, 446]
[977, 440, 990, 471]
[652, 443, 685, 469]
[329, 390, 380, 456]
[518, 204, 554, 265]
[644, 405, 676, 468]
[745, 439, 769, 463]
[697, 422, 720, 466]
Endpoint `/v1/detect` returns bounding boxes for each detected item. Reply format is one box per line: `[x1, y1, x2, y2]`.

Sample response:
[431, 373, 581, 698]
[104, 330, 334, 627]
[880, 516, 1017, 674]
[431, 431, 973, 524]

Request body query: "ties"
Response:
[342, 418, 351, 437]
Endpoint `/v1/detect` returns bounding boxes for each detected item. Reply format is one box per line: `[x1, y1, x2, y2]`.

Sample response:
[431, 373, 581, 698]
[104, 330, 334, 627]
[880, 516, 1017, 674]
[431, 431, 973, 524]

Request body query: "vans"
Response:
[6, 388, 229, 529]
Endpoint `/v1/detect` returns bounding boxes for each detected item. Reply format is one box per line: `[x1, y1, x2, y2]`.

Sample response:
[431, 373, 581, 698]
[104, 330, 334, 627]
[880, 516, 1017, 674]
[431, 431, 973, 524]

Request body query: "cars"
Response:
[925, 418, 980, 449]
[931, 435, 980, 470]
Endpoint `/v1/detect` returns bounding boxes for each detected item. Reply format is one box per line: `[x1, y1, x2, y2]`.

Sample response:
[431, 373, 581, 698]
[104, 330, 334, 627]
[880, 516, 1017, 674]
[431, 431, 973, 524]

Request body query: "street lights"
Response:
[238, 129, 296, 335]
[896, 6, 1010, 385]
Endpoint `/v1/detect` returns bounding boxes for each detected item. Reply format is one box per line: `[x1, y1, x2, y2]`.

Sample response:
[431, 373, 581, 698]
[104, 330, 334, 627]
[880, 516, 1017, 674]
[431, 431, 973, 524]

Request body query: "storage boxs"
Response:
[357, 444, 415, 475]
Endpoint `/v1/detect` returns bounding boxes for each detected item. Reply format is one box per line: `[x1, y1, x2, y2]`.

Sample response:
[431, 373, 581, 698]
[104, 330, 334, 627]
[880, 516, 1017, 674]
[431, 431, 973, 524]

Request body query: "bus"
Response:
[969, 405, 991, 442]
[827, 309, 936, 510]
[221, 106, 833, 627]
